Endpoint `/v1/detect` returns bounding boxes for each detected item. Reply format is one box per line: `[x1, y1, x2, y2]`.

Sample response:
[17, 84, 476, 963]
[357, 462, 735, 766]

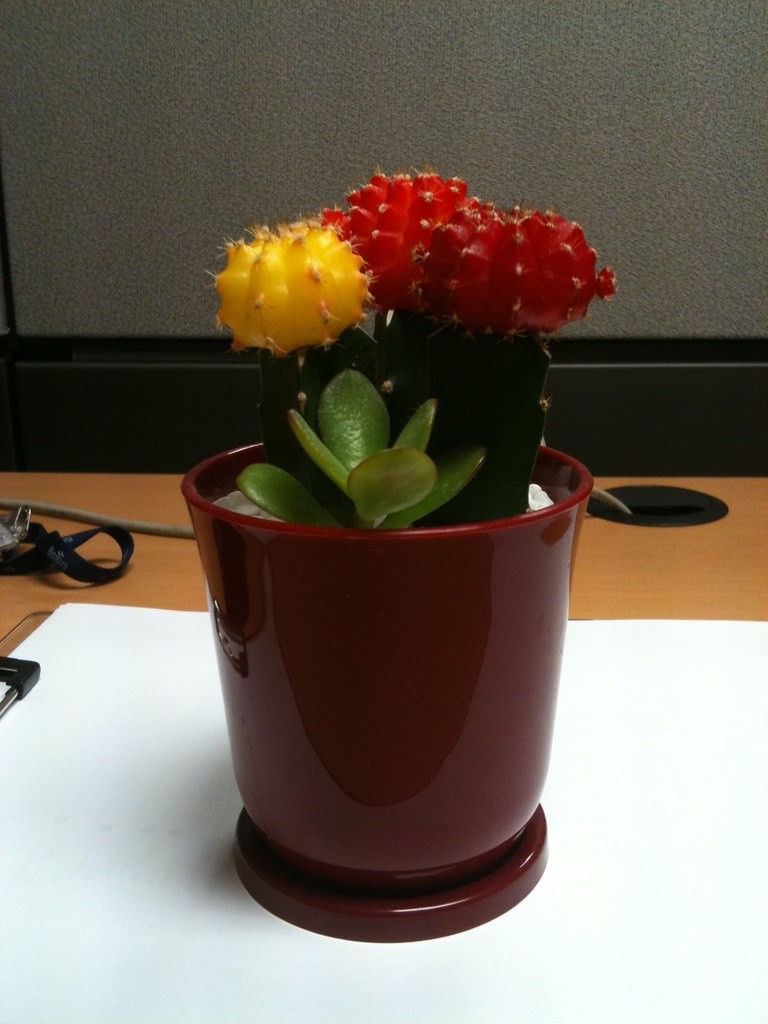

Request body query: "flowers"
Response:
[196, 163, 619, 528]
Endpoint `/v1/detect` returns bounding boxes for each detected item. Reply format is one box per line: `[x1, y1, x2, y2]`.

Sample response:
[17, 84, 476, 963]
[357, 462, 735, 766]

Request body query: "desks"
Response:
[0, 471, 768, 1024]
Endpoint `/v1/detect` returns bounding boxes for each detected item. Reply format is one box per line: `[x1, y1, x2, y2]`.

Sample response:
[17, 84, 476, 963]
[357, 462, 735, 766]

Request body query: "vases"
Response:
[179, 442, 595, 889]
